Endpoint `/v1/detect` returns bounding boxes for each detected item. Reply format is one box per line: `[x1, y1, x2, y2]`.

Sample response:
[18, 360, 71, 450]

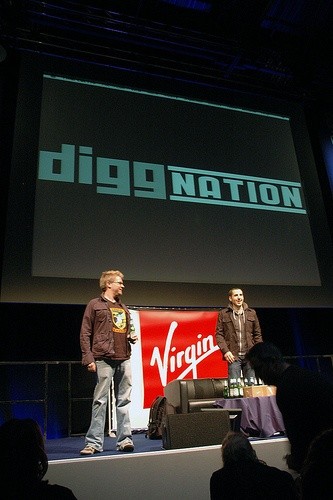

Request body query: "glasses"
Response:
[112, 282, 125, 286]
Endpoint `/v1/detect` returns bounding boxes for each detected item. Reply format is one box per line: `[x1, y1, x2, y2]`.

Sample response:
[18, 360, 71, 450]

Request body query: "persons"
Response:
[296, 431, 333, 499]
[215, 285, 265, 395]
[245, 343, 333, 475]
[210, 431, 292, 499]
[80, 271, 139, 454]
[1, 417, 75, 498]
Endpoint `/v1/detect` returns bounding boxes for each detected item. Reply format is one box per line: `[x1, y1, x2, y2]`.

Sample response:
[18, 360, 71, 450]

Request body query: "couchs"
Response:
[179, 379, 228, 413]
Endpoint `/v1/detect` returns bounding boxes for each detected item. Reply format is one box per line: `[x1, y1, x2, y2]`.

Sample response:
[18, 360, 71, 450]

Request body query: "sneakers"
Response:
[80, 445, 98, 455]
[116, 440, 133, 451]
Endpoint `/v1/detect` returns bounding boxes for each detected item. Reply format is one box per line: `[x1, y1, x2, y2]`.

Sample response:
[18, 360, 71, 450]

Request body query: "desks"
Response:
[213, 395, 287, 438]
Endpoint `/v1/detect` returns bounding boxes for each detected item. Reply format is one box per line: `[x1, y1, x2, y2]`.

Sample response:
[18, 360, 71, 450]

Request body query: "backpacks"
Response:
[147, 395, 176, 440]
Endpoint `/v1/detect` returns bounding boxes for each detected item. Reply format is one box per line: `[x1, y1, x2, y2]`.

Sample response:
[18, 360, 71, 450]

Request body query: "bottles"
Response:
[233, 376, 238, 397]
[230, 378, 234, 397]
[129, 319, 137, 344]
[237, 378, 240, 397]
[223, 379, 229, 398]
[240, 376, 244, 397]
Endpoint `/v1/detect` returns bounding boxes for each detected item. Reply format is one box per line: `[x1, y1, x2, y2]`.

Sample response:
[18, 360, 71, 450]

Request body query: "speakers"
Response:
[162, 411, 231, 450]
